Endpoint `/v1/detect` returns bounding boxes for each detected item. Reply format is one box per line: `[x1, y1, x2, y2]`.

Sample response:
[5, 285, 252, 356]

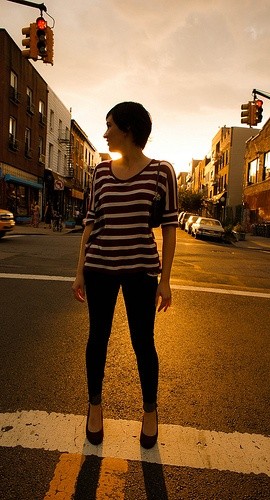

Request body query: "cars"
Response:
[190, 217, 226, 244]
[177, 211, 207, 235]
[0, 209, 15, 238]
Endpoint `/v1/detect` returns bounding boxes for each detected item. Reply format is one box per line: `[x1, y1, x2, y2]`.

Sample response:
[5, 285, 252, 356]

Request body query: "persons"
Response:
[31, 200, 82, 231]
[72, 102, 178, 449]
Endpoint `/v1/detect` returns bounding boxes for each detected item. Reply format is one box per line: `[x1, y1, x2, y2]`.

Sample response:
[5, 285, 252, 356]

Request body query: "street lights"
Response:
[35, 17, 48, 56]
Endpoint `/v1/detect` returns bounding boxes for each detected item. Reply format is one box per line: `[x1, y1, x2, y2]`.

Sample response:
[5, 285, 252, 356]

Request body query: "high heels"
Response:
[139, 409, 158, 450]
[85, 403, 104, 445]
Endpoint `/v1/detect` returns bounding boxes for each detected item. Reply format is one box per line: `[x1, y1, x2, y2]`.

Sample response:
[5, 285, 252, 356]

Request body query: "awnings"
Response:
[211, 192, 226, 205]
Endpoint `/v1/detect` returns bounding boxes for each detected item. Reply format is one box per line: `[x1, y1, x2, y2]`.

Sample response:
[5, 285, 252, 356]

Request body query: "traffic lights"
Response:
[251, 99, 263, 123]
[20, 23, 38, 61]
[240, 101, 252, 125]
